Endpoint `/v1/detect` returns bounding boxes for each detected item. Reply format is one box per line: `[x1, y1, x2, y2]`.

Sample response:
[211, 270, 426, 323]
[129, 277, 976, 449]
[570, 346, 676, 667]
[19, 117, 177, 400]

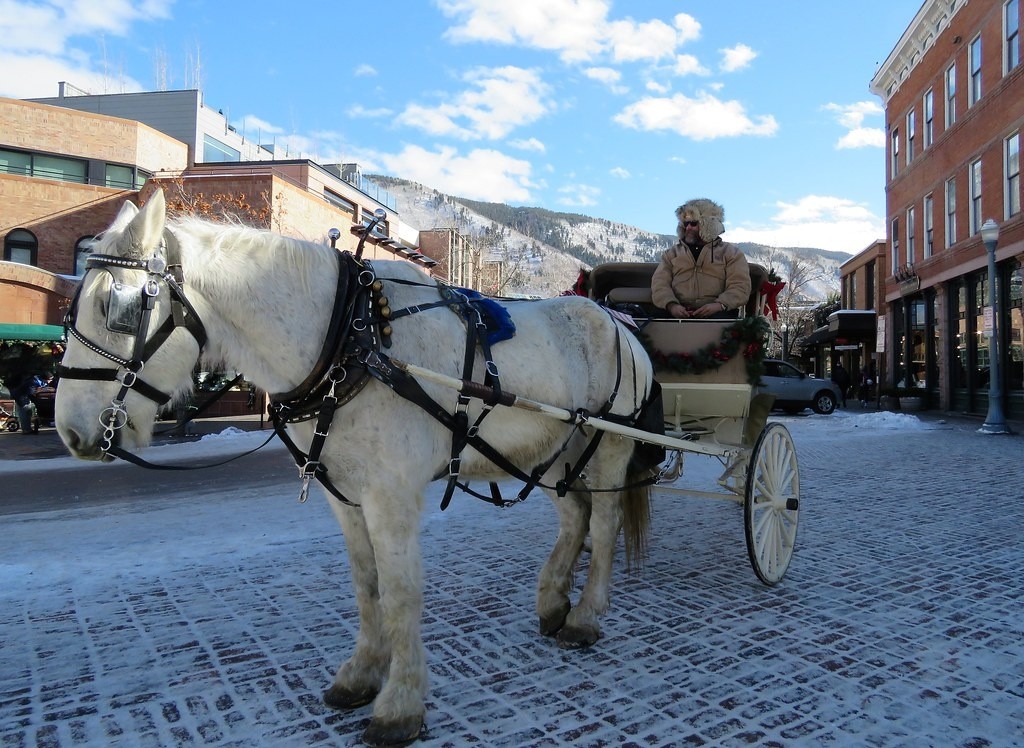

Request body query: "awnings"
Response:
[1, 323, 72, 342]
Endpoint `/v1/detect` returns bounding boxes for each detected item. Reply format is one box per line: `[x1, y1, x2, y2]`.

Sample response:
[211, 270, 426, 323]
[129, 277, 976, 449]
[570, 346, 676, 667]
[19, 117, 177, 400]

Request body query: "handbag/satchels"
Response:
[860, 377, 865, 386]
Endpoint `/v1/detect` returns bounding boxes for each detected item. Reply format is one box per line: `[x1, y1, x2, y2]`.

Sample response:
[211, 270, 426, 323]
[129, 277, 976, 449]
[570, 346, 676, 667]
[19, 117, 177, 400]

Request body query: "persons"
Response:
[857, 370, 869, 409]
[13, 370, 54, 434]
[830, 361, 850, 409]
[650, 198, 753, 320]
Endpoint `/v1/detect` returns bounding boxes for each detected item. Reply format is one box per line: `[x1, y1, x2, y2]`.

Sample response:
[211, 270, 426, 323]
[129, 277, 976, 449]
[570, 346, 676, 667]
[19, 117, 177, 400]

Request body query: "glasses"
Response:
[47, 379, 54, 380]
[684, 221, 698, 226]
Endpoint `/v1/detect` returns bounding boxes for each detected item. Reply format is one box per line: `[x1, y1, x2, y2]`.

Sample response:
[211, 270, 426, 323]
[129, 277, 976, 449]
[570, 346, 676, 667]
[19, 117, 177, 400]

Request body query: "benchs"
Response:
[588, 262, 768, 384]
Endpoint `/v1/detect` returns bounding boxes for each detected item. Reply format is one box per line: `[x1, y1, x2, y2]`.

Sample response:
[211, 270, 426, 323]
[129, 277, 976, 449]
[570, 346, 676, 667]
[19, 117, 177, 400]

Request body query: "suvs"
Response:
[759, 357, 844, 414]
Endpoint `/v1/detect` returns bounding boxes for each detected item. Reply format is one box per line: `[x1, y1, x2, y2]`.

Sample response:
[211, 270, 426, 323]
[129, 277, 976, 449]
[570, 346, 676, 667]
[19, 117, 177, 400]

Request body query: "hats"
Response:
[674, 199, 725, 243]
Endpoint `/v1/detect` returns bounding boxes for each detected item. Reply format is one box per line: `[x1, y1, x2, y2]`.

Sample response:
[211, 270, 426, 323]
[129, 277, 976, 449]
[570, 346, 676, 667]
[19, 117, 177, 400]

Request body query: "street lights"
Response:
[976, 216, 1012, 435]
[780, 323, 788, 362]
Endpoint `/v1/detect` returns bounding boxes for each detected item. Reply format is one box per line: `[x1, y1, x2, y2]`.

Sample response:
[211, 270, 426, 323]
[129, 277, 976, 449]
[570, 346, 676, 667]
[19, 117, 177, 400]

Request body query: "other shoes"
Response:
[22, 430, 35, 434]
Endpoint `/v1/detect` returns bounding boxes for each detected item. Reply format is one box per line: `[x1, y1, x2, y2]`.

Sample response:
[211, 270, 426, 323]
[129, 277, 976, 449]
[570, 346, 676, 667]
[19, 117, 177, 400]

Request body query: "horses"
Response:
[53, 187, 654, 747]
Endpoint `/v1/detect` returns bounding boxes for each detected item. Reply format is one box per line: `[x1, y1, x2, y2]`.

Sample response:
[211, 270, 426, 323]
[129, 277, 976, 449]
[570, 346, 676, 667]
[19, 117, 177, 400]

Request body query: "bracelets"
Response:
[721, 302, 727, 311]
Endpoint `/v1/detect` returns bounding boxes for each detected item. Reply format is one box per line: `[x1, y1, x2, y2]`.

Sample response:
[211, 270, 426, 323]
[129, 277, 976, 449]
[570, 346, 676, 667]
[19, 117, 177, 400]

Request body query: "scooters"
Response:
[0, 407, 20, 433]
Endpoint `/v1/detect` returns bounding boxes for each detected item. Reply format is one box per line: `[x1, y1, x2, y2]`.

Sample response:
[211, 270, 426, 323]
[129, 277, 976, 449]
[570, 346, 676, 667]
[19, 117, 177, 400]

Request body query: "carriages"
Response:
[53, 186, 800, 747]
